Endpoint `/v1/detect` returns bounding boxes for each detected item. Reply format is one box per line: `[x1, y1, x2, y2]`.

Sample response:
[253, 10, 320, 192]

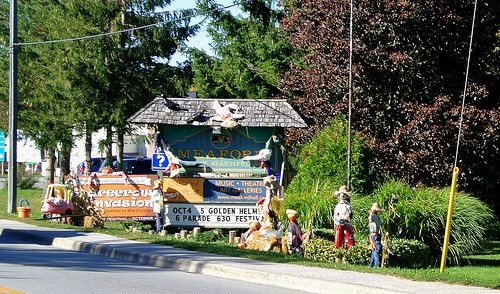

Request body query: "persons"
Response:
[286, 208, 304, 252]
[151, 180, 176, 231]
[334, 184, 356, 248]
[236, 219, 262, 248]
[92, 167, 128, 181]
[367, 202, 383, 267]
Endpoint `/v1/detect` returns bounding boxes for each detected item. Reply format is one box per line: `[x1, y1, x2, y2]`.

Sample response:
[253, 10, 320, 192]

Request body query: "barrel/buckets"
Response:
[17, 198, 31, 218]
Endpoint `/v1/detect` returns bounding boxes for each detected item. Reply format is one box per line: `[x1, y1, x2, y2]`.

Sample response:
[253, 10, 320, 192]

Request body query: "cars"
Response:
[76, 156, 157, 175]
[40, 183, 75, 219]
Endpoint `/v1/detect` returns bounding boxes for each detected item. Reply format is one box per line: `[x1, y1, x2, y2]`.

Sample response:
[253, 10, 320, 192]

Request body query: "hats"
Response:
[334, 185, 351, 197]
[368, 202, 381, 213]
[286, 209, 300, 218]
